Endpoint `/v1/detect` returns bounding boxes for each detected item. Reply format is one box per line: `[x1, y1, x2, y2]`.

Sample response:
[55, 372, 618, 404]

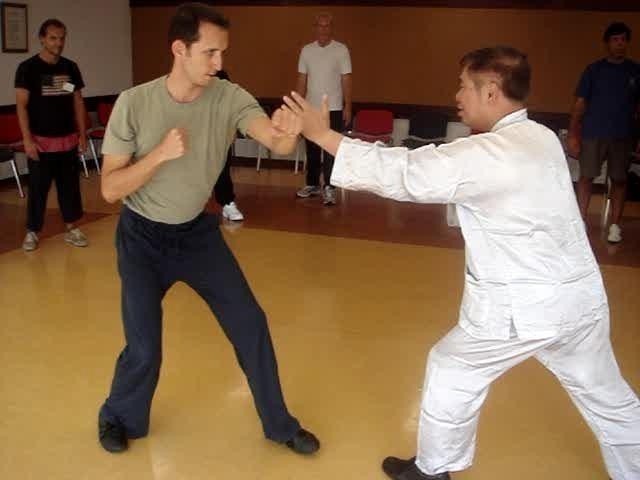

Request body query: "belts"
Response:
[127, 208, 206, 231]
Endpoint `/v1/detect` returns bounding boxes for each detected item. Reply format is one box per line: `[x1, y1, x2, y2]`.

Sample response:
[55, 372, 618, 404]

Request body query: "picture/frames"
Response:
[1, 2, 29, 53]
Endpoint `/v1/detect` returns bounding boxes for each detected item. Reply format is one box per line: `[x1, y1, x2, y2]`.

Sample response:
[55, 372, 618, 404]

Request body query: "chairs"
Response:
[0, 149, 24, 198]
[400, 111, 449, 149]
[87, 112, 104, 175]
[352, 109, 394, 144]
[256, 103, 307, 175]
[99, 104, 113, 125]
[0, 114, 25, 153]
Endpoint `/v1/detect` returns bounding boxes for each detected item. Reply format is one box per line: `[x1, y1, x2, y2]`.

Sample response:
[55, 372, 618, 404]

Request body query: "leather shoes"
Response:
[96, 408, 129, 453]
[381, 455, 450, 480]
[262, 425, 321, 455]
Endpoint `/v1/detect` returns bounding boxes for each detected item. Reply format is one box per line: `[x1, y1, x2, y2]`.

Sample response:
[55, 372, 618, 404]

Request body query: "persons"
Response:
[97, 6, 322, 455]
[14, 19, 90, 252]
[280, 45, 639, 480]
[211, 69, 243, 221]
[567, 22, 639, 243]
[296, 12, 352, 207]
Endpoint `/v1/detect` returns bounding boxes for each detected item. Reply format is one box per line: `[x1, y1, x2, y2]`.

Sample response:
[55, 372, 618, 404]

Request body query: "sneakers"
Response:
[606, 222, 622, 243]
[321, 184, 337, 207]
[221, 199, 244, 220]
[23, 231, 39, 252]
[63, 227, 89, 247]
[295, 185, 322, 199]
